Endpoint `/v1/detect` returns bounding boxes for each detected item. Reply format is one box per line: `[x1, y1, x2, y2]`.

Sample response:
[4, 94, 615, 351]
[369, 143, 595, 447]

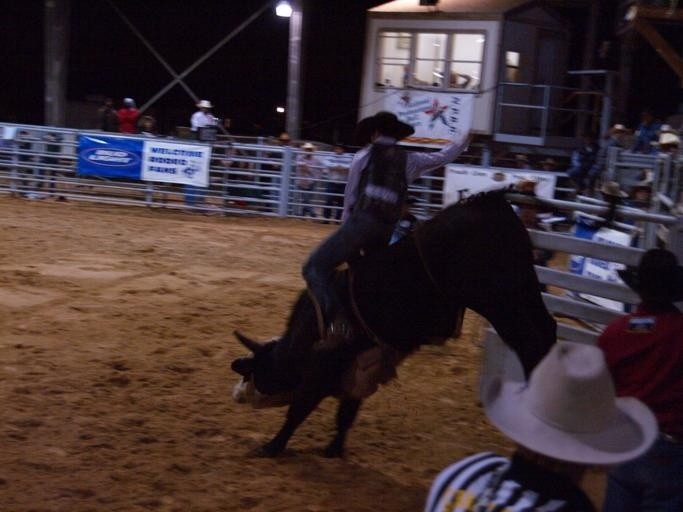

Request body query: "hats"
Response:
[596, 124, 680, 198]
[480, 338, 659, 466]
[301, 142, 316, 149]
[359, 111, 415, 141]
[617, 248, 683, 303]
[278, 132, 291, 141]
[196, 100, 214, 108]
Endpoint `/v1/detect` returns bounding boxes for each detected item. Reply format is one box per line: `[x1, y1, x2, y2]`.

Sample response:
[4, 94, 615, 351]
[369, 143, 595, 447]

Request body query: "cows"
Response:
[230, 184, 556, 456]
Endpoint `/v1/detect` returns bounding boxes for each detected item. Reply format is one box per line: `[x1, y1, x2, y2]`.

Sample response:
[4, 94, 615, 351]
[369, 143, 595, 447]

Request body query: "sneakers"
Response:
[312, 316, 353, 353]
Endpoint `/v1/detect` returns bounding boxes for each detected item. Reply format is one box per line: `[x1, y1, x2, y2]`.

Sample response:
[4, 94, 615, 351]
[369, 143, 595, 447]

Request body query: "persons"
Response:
[96, 96, 353, 226]
[402, 66, 427, 86]
[450, 73, 470, 89]
[595, 247, 683, 511]
[301, 93, 477, 338]
[15, 132, 33, 196]
[425, 338, 660, 510]
[483, 106, 682, 306]
[36, 134, 60, 197]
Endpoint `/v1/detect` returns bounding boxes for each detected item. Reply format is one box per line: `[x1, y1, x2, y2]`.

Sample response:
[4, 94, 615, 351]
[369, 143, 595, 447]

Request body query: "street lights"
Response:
[273, 1, 304, 140]
